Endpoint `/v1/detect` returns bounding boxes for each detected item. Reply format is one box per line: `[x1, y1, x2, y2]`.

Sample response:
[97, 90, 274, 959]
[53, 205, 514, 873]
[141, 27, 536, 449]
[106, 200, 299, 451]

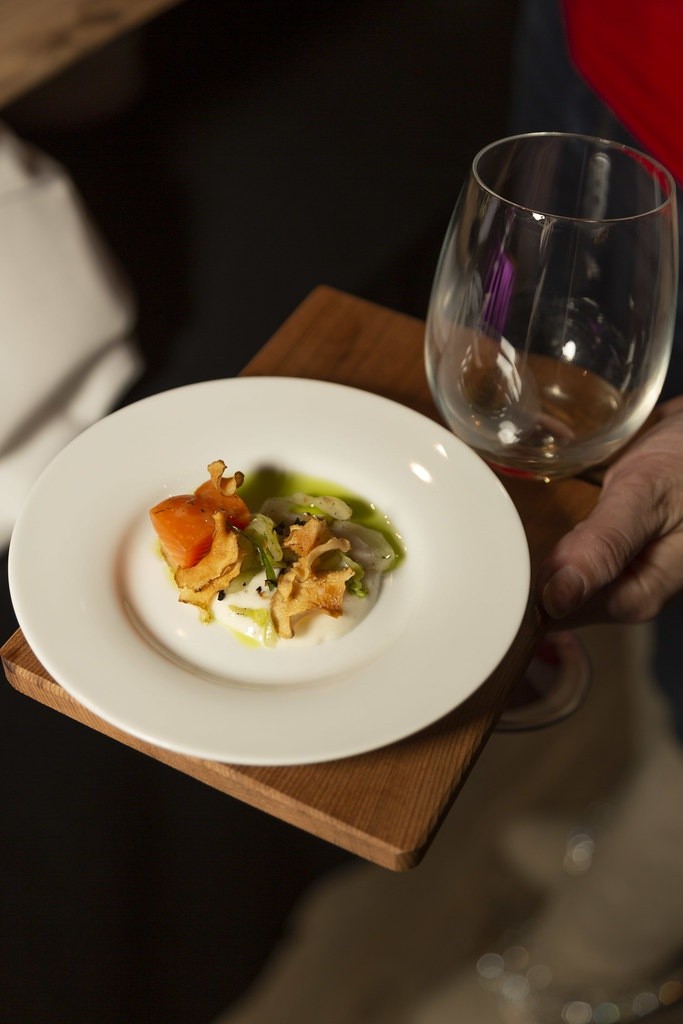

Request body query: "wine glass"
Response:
[424, 130, 678, 729]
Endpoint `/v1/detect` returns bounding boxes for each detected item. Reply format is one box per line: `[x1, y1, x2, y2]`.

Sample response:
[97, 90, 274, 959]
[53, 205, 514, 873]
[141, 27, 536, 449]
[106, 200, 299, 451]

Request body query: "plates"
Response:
[7, 377, 530, 766]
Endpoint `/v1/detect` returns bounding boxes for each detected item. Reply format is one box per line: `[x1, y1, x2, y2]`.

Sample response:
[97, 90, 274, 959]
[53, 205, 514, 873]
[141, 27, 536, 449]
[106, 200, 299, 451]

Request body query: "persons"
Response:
[221, 0, 683, 1022]
